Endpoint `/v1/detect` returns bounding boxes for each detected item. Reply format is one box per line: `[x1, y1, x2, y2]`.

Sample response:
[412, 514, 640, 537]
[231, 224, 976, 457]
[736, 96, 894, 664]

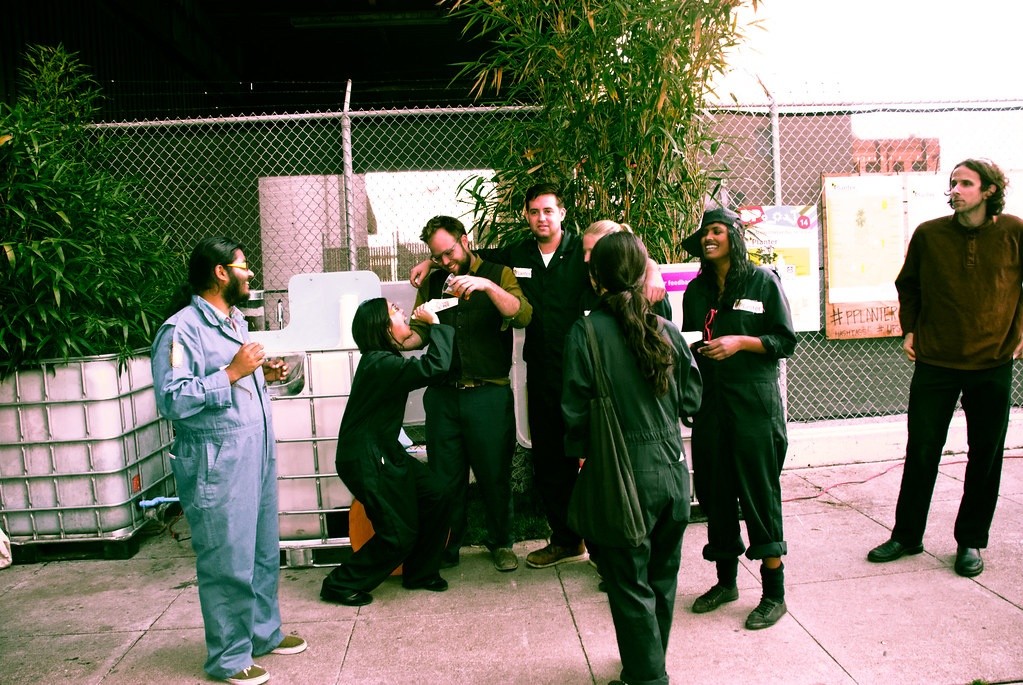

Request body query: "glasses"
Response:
[221, 257, 249, 271]
[431, 236, 464, 264]
[703, 308, 717, 343]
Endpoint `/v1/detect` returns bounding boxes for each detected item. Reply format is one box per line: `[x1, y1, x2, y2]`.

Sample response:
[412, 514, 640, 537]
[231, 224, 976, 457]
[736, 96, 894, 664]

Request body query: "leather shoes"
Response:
[866, 538, 922, 563]
[954, 543, 983, 576]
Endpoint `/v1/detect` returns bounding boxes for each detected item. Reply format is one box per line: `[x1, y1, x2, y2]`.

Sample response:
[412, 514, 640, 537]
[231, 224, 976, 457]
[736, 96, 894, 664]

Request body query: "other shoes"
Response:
[320, 586, 372, 606]
[608, 680, 626, 685]
[440, 558, 459, 570]
[430, 576, 449, 591]
[491, 546, 519, 572]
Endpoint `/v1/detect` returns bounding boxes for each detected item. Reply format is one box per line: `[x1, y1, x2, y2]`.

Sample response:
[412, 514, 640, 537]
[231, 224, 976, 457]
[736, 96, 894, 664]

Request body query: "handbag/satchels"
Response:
[566, 317, 645, 549]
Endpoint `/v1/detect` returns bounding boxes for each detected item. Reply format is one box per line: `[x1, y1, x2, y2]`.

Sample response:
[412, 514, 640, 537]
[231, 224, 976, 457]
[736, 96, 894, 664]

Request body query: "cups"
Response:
[442, 273, 474, 301]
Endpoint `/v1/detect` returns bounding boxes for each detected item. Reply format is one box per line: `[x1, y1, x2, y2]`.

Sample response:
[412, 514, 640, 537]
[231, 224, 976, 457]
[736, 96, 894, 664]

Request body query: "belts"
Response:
[452, 379, 489, 389]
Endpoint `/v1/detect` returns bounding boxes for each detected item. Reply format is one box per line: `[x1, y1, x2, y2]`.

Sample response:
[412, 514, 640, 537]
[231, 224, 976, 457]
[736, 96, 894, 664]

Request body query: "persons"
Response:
[680, 208, 798, 630]
[583, 220, 672, 592]
[401, 215, 533, 571]
[151, 236, 307, 685]
[560, 231, 702, 685]
[409, 182, 666, 568]
[319, 298, 456, 605]
[867, 156, 1023, 575]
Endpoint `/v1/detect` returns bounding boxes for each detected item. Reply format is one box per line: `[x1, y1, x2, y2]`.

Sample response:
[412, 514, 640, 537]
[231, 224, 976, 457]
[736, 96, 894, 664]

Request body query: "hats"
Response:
[681, 206, 745, 257]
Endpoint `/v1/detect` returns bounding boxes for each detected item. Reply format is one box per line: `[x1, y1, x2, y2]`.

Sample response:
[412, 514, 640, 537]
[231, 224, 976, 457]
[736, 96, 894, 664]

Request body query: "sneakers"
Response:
[526, 539, 588, 568]
[223, 664, 270, 684]
[587, 552, 604, 581]
[270, 636, 307, 653]
[745, 597, 784, 629]
[692, 583, 739, 614]
